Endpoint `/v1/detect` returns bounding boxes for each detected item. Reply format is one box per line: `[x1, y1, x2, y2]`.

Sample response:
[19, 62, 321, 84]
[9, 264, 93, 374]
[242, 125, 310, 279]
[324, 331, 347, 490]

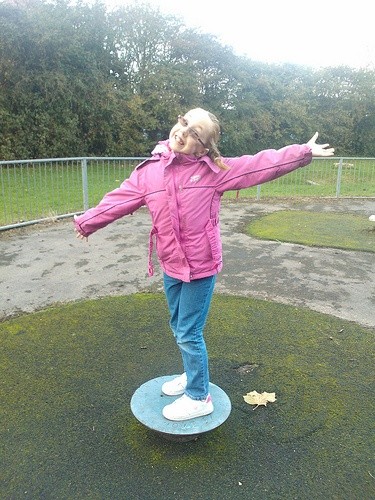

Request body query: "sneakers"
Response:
[161, 371, 188, 396]
[163, 394, 215, 421]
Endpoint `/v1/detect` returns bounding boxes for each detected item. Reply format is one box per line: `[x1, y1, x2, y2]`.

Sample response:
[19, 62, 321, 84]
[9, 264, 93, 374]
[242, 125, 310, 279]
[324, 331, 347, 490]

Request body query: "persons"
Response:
[74, 107, 335, 422]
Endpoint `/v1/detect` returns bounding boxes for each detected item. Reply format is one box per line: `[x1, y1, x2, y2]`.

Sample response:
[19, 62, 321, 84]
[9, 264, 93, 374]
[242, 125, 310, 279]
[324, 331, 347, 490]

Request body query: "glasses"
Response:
[178, 115, 207, 148]
